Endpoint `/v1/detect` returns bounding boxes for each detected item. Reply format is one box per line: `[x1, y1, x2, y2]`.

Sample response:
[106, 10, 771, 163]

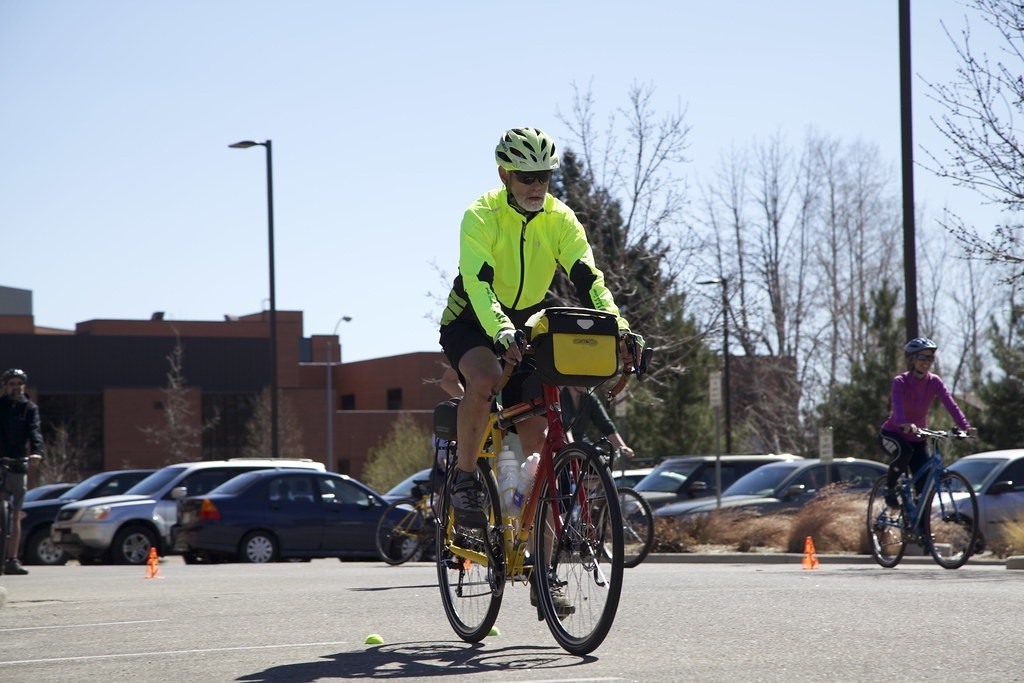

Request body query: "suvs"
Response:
[51, 460, 327, 567]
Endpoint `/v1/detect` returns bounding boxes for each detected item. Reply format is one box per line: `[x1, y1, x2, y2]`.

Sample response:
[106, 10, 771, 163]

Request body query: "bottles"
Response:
[147, 547, 161, 578]
[511, 453, 540, 517]
[496, 449, 521, 505]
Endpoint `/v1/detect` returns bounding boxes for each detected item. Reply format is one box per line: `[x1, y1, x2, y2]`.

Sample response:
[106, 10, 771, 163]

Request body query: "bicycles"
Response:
[429, 308, 655, 656]
[865, 426, 983, 570]
[0, 456, 43, 576]
[375, 471, 655, 572]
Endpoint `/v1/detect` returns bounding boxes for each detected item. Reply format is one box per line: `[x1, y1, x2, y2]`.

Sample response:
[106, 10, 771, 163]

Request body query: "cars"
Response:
[619, 456, 802, 528]
[651, 459, 917, 527]
[169, 468, 440, 564]
[363, 468, 446, 518]
[22, 482, 119, 501]
[586, 469, 686, 501]
[17, 469, 162, 567]
[928, 449, 1024, 552]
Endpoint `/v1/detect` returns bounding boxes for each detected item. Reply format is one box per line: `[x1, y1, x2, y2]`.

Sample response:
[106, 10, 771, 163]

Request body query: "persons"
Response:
[440, 124, 645, 614]
[559, 384, 634, 515]
[441, 364, 525, 581]
[429, 433, 456, 516]
[0, 369, 43, 575]
[879, 338, 977, 546]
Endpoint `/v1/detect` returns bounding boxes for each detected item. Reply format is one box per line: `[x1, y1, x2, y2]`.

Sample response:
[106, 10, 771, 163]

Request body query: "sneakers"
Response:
[449, 468, 488, 528]
[529, 574, 576, 613]
[2, 559, 28, 575]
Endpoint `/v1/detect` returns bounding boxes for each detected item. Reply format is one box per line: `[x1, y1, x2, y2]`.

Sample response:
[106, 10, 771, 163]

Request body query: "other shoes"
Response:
[883, 488, 899, 508]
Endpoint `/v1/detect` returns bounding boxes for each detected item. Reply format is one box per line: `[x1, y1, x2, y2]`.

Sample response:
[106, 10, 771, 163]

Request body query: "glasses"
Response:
[915, 352, 935, 362]
[515, 169, 553, 185]
[5, 381, 24, 387]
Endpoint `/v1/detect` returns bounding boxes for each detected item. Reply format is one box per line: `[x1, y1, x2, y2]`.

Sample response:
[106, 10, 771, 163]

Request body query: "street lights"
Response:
[326, 316, 351, 470]
[228, 139, 280, 459]
[695, 277, 733, 456]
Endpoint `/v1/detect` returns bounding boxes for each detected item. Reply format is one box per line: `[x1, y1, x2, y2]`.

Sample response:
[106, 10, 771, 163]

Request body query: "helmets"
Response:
[0, 369, 28, 382]
[904, 338, 938, 353]
[495, 127, 560, 171]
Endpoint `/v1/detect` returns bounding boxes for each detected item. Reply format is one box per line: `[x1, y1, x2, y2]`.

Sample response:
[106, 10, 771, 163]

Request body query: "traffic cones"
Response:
[801, 536, 820, 570]
[142, 547, 164, 579]
